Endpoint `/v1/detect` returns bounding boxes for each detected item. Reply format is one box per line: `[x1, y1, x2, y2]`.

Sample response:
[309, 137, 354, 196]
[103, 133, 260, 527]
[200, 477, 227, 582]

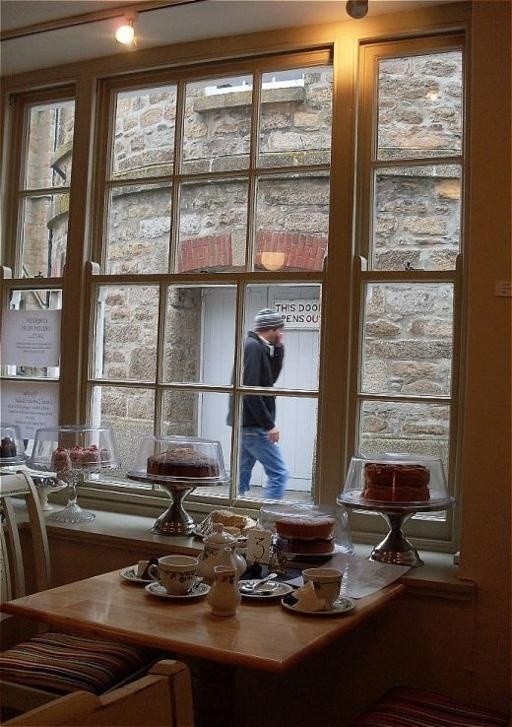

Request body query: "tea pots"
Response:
[199, 525, 247, 577]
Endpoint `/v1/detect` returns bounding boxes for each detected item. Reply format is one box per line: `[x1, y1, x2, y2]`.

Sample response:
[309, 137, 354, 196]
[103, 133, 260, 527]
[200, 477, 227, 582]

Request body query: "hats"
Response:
[254, 308, 285, 332]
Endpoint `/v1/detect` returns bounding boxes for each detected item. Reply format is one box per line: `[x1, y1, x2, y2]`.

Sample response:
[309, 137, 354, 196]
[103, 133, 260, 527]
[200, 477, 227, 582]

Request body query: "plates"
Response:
[119, 567, 204, 585]
[335, 491, 457, 567]
[0, 452, 27, 466]
[241, 582, 293, 602]
[281, 595, 356, 617]
[32, 483, 68, 511]
[126, 468, 231, 537]
[240, 581, 278, 593]
[144, 581, 212, 599]
[273, 552, 335, 570]
[25, 457, 121, 523]
[192, 527, 252, 541]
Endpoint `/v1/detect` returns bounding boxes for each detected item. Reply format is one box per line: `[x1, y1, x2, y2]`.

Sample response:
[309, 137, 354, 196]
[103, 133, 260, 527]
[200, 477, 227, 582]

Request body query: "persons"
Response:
[224, 308, 288, 498]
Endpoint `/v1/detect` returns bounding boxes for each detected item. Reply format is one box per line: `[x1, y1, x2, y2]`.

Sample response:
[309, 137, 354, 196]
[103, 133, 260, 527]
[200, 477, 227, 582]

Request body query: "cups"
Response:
[302, 568, 343, 608]
[148, 555, 198, 595]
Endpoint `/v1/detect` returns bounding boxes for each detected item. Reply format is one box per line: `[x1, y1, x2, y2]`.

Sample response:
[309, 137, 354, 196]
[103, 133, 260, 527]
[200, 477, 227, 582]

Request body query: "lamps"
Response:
[115, 11, 139, 44]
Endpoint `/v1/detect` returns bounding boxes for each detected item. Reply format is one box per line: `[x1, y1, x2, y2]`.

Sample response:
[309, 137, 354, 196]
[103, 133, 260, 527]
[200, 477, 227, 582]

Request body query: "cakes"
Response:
[148, 447, 220, 478]
[1, 438, 16, 458]
[212, 510, 246, 535]
[84, 443, 101, 469]
[71, 445, 83, 469]
[99, 448, 111, 468]
[363, 463, 430, 501]
[276, 515, 335, 539]
[276, 538, 335, 554]
[50, 447, 71, 472]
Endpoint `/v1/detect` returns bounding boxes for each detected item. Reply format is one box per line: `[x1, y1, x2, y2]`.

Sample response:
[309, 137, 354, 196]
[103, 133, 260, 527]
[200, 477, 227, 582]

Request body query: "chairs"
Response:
[355, 685, 510, 727]
[0, 469, 157, 713]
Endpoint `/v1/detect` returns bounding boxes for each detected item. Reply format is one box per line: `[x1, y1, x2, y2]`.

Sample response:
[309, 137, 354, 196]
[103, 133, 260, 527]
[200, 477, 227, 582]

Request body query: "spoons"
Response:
[241, 573, 275, 593]
